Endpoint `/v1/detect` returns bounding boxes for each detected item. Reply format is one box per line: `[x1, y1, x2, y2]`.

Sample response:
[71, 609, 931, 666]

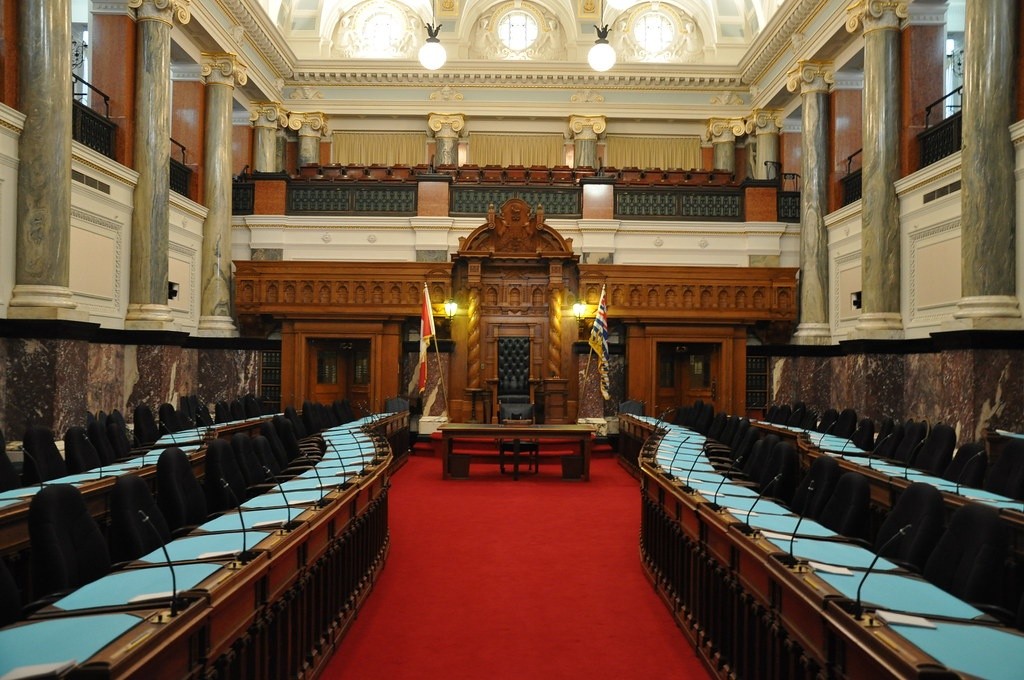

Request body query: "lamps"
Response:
[444, 298, 457, 328]
[573, 298, 588, 324]
[588, 0, 616, 72]
[418, 0, 446, 70]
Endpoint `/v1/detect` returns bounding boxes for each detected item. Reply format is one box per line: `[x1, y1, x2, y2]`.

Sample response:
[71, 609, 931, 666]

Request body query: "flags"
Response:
[589, 284, 610, 400]
[417, 288, 435, 395]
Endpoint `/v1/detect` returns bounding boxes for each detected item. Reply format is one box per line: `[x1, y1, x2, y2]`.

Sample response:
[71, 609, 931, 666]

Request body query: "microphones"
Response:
[17, 391, 396, 619]
[624, 400, 984, 621]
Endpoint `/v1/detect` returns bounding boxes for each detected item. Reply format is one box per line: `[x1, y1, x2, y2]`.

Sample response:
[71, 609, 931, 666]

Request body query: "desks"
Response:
[0, 407, 305, 554]
[617, 413, 1024, 680]
[727, 416, 1024, 547]
[438, 424, 596, 483]
[0, 410, 409, 680]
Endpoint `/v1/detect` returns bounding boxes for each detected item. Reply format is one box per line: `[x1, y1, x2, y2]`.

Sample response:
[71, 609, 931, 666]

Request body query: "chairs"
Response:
[617, 401, 644, 417]
[673, 401, 1024, 636]
[499, 405, 539, 476]
[0, 392, 424, 629]
